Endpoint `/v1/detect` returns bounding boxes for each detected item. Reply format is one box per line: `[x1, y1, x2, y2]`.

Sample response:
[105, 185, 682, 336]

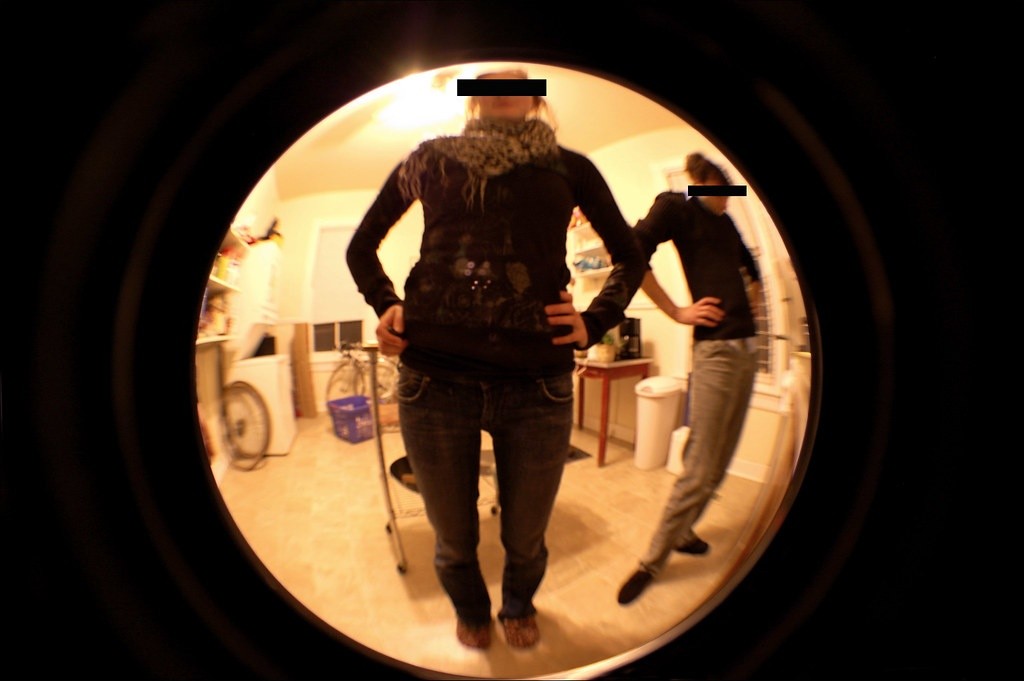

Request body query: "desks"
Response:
[575, 358, 654, 468]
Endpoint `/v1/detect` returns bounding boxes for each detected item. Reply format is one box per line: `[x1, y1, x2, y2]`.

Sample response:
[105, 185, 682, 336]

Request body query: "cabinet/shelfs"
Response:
[569, 222, 618, 280]
[195, 225, 252, 346]
[309, 348, 402, 416]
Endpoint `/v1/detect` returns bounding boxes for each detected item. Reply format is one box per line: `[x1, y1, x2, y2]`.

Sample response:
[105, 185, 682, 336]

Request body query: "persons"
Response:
[345, 68, 640, 650]
[617, 150, 759, 604]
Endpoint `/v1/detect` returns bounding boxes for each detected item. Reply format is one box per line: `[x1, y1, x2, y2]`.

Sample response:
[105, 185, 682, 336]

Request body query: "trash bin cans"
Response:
[634, 375, 682, 471]
[327, 396, 374, 445]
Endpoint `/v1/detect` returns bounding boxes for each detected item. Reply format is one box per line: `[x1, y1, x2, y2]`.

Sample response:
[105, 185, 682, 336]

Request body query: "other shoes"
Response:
[673, 537, 711, 555]
[618, 570, 652, 604]
[455, 619, 493, 648]
[502, 613, 539, 648]
